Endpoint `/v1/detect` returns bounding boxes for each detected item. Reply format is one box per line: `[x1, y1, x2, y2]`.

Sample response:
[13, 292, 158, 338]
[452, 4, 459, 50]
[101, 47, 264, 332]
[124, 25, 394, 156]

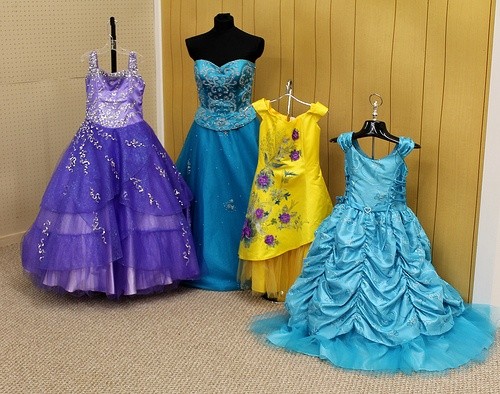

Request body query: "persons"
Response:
[174, 12, 265, 292]
